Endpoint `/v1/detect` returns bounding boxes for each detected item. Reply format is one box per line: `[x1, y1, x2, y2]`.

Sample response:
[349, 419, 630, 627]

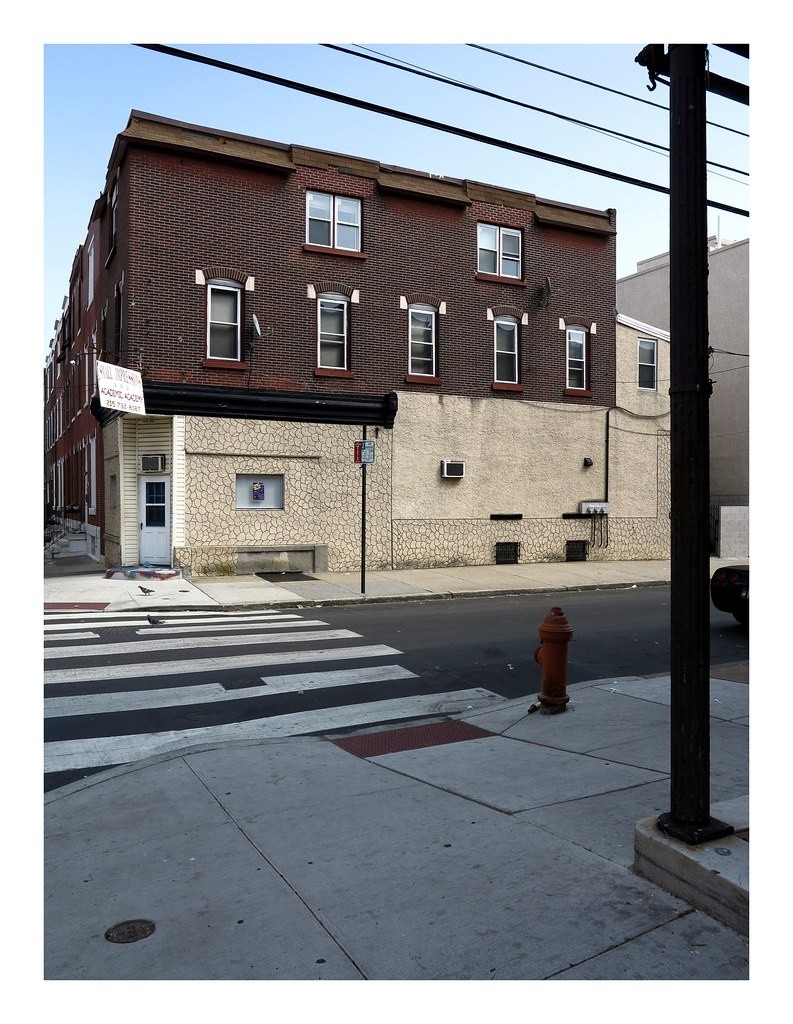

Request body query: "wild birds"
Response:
[138, 585, 155, 596]
[147, 614, 166, 628]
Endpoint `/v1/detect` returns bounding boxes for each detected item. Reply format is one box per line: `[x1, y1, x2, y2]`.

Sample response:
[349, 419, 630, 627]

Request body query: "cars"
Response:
[710, 564, 749, 625]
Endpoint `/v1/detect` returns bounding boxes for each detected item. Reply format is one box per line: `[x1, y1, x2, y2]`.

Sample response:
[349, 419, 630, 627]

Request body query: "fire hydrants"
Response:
[534, 606, 575, 715]
